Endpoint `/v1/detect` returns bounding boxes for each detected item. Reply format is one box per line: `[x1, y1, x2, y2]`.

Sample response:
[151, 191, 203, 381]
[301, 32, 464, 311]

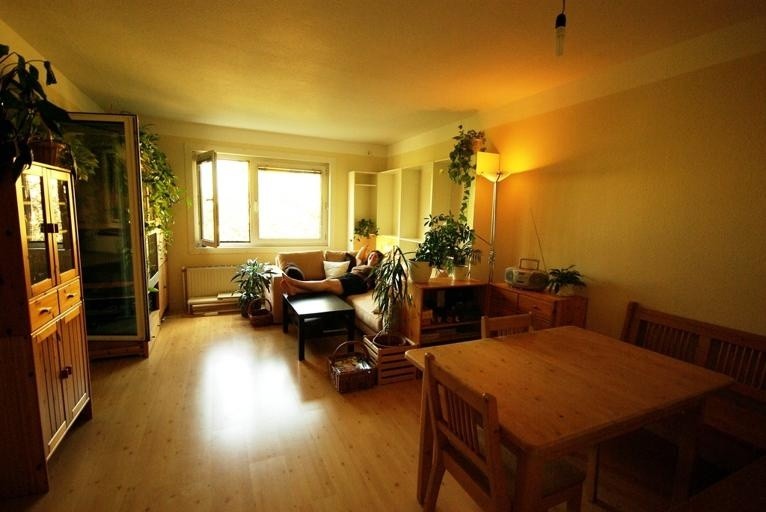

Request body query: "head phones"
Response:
[545, 279, 560, 294]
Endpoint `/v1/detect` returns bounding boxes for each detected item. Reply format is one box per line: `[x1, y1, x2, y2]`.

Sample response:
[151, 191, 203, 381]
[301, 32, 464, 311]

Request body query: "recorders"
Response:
[505, 259, 550, 291]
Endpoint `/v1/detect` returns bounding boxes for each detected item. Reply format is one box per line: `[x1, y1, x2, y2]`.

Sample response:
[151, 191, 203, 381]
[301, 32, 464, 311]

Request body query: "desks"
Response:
[403, 325, 735, 512]
[280, 292, 355, 362]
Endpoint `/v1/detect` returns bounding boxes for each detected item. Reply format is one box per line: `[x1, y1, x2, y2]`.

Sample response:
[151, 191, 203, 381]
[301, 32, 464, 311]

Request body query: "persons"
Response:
[280, 245, 384, 297]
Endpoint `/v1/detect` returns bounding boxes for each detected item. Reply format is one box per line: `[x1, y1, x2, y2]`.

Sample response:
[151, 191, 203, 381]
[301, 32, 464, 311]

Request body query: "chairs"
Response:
[479, 311, 534, 341]
[417, 349, 587, 512]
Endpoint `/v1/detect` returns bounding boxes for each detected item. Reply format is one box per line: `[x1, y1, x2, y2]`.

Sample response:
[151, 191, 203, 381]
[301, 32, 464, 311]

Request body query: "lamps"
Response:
[478, 170, 513, 281]
[551, 1, 571, 54]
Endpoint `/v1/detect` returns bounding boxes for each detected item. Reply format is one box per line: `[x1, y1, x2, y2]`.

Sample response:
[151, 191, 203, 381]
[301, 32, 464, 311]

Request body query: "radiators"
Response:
[180, 262, 265, 314]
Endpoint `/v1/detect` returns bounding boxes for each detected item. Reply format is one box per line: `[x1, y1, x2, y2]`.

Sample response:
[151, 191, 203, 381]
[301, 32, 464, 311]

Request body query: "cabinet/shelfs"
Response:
[73, 148, 170, 362]
[488, 279, 589, 334]
[402, 278, 492, 350]
[345, 149, 501, 280]
[0, 156, 94, 499]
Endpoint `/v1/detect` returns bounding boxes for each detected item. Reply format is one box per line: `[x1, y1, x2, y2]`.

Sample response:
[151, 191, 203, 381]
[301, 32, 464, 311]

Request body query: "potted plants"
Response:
[228, 256, 274, 318]
[403, 226, 449, 284]
[27, 118, 101, 182]
[367, 244, 418, 386]
[446, 124, 487, 190]
[544, 265, 587, 299]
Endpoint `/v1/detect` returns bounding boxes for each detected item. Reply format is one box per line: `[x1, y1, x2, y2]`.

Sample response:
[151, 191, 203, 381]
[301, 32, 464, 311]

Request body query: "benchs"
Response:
[618, 300, 764, 459]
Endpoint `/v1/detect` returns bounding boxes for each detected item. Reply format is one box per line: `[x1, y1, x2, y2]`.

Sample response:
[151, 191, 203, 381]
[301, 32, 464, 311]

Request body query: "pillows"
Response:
[322, 260, 350, 279]
[282, 262, 305, 281]
[343, 252, 367, 273]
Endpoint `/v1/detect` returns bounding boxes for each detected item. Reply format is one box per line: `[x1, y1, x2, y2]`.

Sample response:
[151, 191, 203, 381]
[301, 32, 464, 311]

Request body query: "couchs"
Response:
[260, 249, 409, 339]
